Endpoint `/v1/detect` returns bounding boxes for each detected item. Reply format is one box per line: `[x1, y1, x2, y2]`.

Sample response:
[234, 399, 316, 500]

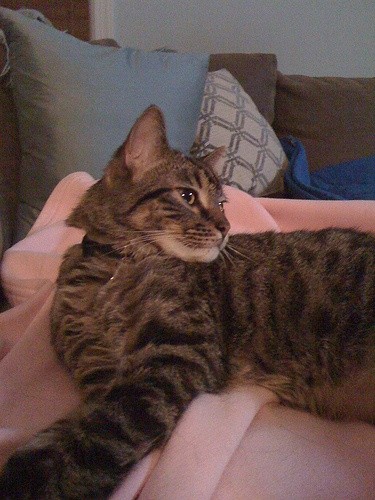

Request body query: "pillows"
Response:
[0, 9, 291, 246]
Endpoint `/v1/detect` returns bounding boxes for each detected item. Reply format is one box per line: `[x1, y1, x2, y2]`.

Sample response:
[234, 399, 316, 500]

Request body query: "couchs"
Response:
[0, 73, 375, 500]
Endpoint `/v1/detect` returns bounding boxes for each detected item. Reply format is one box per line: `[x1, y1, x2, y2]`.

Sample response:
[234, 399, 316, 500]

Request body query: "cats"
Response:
[0, 105, 375, 500]
